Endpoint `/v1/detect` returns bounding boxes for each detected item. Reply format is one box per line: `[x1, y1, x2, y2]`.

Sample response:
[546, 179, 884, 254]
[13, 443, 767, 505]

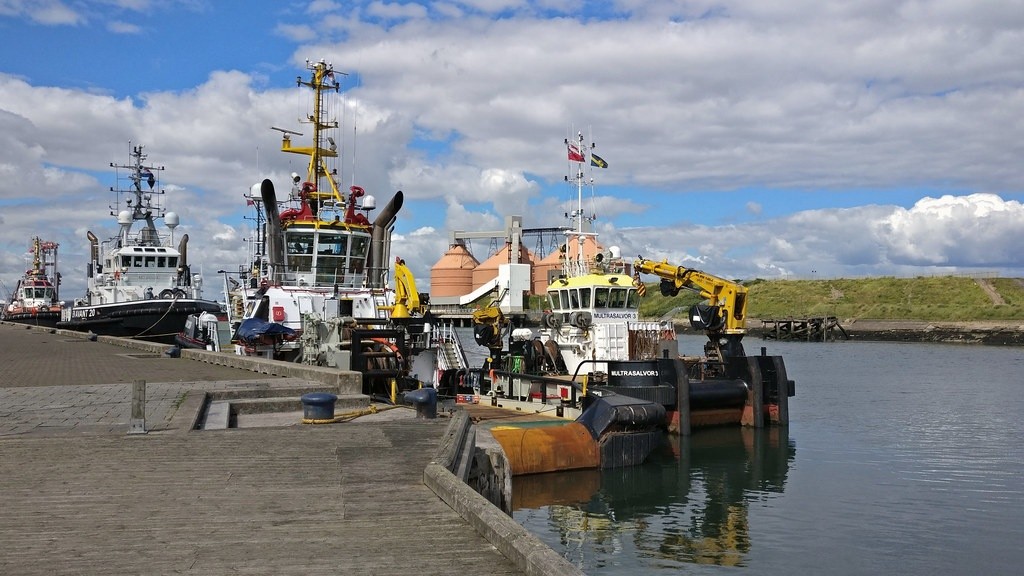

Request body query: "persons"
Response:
[145, 288, 155, 299]
[254, 265, 260, 277]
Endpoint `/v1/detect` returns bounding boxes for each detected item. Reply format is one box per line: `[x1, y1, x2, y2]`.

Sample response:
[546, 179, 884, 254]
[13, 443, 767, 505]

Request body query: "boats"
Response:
[173, 57, 796, 439]
[56, 139, 222, 342]
[0, 234, 66, 329]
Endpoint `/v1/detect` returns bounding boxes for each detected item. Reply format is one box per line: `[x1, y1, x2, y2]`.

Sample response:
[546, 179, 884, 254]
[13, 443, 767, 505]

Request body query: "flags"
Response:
[568, 144, 585, 163]
[591, 153, 608, 168]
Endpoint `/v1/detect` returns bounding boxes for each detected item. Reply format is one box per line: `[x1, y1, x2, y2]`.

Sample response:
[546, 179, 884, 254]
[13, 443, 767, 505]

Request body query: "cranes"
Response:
[634, 255, 748, 376]
